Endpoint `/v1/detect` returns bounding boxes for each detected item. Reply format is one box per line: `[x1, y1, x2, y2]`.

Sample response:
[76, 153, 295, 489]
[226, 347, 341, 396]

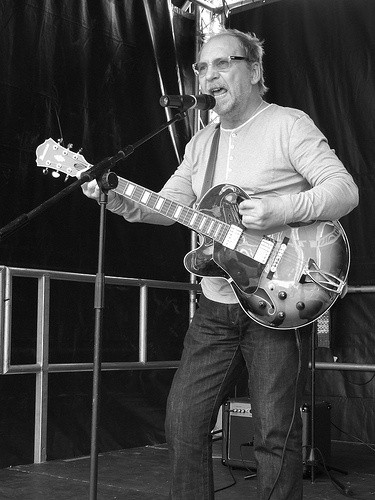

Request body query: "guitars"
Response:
[35, 137, 351, 331]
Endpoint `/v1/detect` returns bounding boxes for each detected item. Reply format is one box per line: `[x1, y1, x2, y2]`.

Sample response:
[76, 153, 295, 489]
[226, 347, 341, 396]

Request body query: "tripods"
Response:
[244, 322, 353, 496]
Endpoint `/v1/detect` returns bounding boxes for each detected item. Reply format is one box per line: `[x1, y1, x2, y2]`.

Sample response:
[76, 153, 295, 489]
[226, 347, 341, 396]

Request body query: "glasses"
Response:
[192, 56, 255, 76]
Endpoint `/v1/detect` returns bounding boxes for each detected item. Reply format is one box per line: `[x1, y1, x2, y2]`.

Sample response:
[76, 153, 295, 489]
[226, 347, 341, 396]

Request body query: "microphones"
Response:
[159, 94, 216, 111]
